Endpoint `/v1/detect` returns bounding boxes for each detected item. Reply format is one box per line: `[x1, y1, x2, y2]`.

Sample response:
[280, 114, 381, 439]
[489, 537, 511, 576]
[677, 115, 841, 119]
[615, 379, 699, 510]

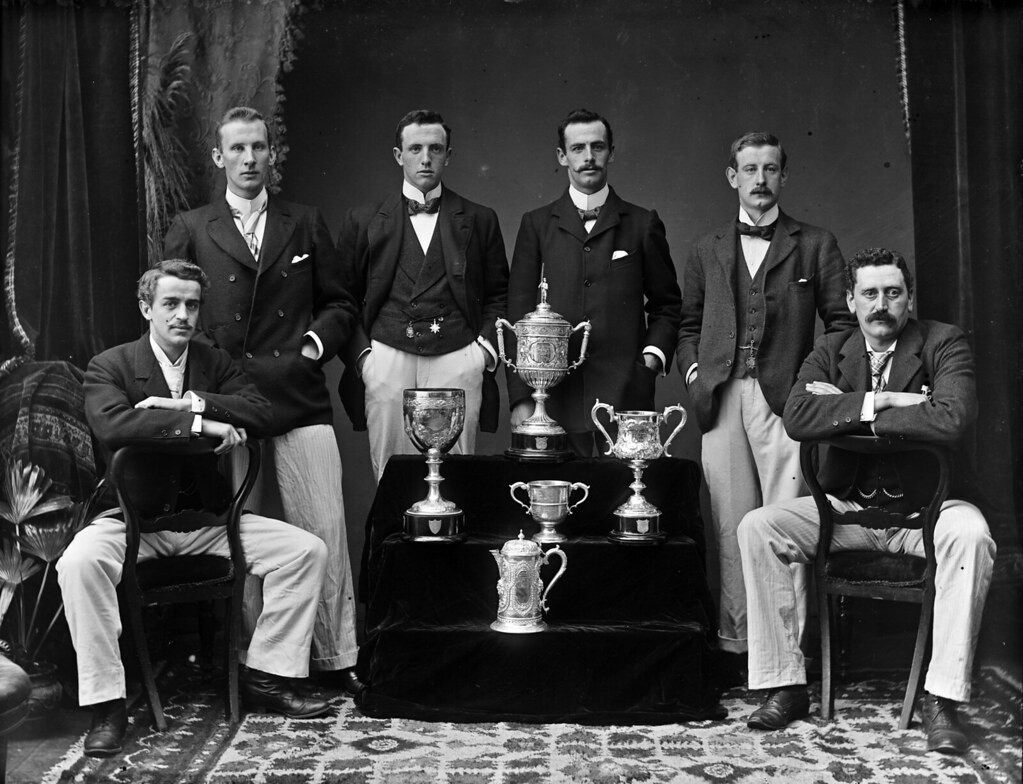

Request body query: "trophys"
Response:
[591, 398, 687, 542]
[495, 277, 591, 457]
[509, 480, 590, 543]
[489, 529, 566, 633]
[402, 387, 465, 542]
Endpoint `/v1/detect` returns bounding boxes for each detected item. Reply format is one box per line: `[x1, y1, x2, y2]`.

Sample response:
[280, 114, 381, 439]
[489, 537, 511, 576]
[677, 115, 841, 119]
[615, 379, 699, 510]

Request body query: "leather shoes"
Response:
[746, 683, 811, 731]
[923, 693, 967, 751]
[322, 664, 365, 695]
[243, 662, 332, 718]
[84, 695, 125, 757]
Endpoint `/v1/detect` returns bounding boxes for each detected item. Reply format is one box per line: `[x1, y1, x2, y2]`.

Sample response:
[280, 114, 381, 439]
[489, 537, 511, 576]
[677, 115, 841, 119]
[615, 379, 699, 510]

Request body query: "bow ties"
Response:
[407, 198, 440, 217]
[735, 220, 778, 241]
[578, 206, 603, 222]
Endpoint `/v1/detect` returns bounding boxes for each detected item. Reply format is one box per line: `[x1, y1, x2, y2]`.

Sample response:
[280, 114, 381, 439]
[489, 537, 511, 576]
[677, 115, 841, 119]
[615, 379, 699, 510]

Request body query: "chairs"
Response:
[797, 427, 950, 729]
[63, 425, 260, 730]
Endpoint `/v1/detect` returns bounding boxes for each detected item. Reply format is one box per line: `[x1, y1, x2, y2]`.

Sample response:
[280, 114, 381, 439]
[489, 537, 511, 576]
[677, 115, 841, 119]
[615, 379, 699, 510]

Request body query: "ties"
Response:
[867, 350, 893, 393]
[229, 200, 270, 262]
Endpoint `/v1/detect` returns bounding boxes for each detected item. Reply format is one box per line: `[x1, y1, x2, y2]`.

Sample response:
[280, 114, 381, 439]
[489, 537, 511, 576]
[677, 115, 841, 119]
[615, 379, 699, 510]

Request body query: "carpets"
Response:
[39, 651, 1023, 782]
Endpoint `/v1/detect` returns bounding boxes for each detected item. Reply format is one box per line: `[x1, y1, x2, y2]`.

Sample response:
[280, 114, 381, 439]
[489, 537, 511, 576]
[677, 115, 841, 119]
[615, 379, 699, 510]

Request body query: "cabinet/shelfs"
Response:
[356, 453, 730, 722]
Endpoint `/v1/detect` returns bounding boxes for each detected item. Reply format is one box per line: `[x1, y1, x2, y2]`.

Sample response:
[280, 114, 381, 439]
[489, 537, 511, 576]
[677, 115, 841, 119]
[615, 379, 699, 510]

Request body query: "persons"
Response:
[56, 261, 330, 756]
[737, 245, 999, 752]
[335, 109, 509, 479]
[675, 133, 849, 685]
[504, 107, 681, 456]
[161, 106, 365, 695]
